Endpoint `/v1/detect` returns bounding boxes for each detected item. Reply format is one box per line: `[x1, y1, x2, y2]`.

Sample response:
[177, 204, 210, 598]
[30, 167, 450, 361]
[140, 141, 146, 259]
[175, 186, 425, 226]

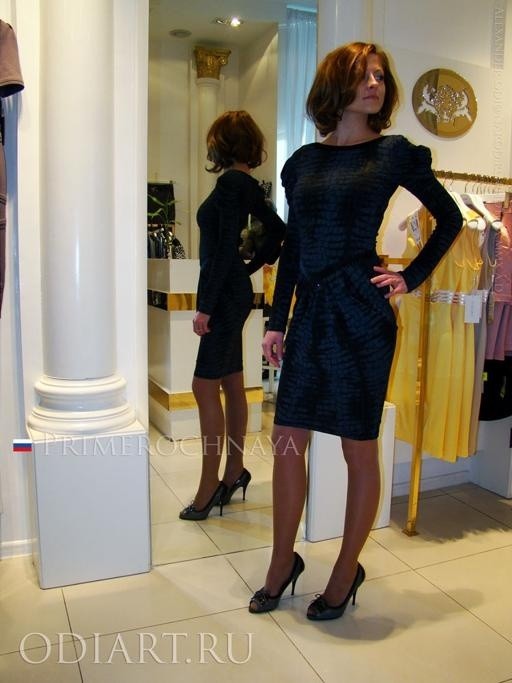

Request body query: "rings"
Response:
[195, 328, 200, 334]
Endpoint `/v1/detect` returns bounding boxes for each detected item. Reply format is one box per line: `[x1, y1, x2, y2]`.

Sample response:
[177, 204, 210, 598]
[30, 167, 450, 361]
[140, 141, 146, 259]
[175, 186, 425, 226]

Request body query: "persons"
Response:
[177, 108, 289, 520]
[245, 41, 466, 621]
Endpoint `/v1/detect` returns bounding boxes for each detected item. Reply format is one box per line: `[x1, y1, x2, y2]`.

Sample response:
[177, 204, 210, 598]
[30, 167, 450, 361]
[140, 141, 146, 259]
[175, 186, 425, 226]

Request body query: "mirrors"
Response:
[149, 4, 320, 578]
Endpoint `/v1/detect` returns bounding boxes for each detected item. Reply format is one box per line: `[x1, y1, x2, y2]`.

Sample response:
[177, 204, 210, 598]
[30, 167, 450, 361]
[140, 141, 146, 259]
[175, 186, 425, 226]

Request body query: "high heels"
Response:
[306, 560, 366, 622]
[248, 551, 305, 614]
[179, 480, 224, 521]
[217, 467, 252, 506]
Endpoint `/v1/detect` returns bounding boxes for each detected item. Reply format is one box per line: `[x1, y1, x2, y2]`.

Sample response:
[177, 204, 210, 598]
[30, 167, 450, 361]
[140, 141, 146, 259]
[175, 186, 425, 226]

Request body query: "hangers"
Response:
[397, 168, 511, 234]
[150, 218, 174, 242]
